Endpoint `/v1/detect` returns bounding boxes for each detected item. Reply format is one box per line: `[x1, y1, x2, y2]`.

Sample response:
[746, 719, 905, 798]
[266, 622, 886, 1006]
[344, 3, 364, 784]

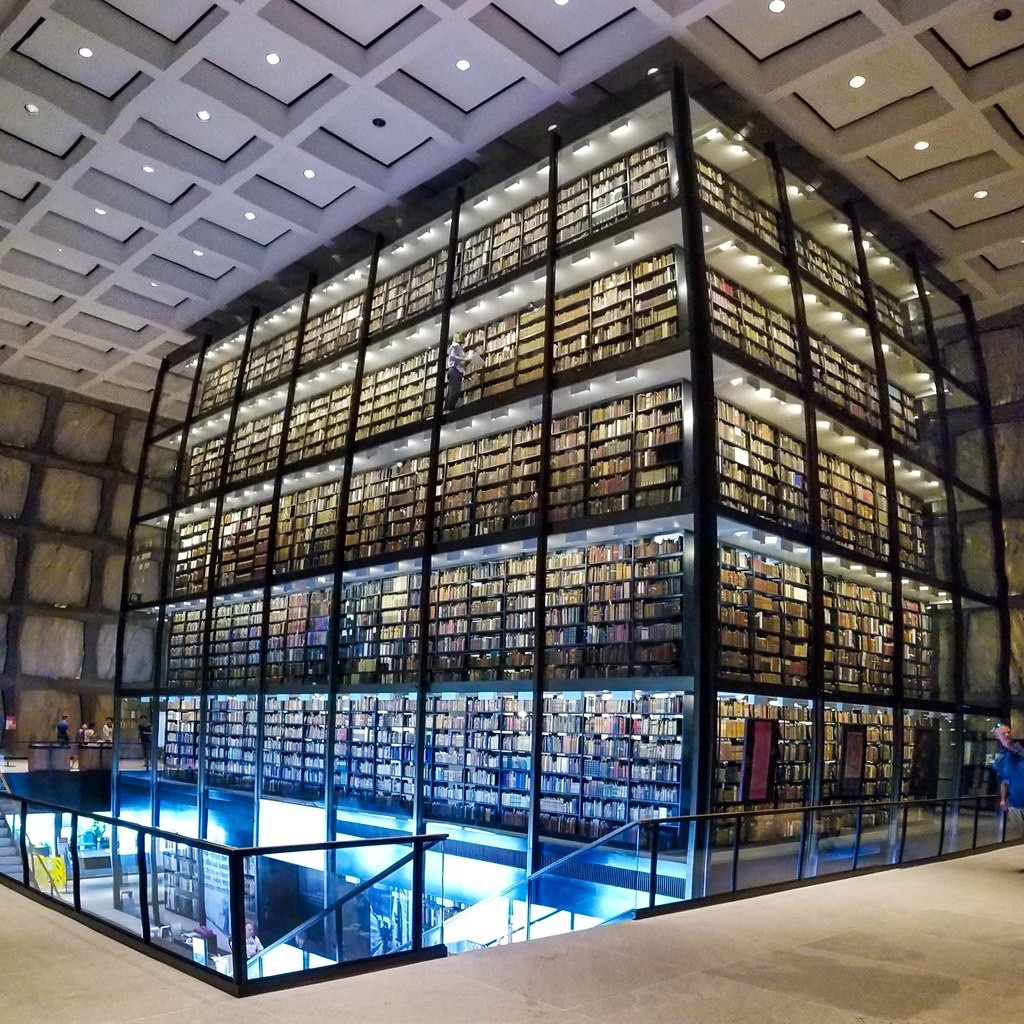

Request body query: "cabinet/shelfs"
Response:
[697, 150, 940, 854]
[422, 892, 471, 934]
[157, 130, 695, 852]
[161, 839, 202, 923]
[242, 856, 298, 948]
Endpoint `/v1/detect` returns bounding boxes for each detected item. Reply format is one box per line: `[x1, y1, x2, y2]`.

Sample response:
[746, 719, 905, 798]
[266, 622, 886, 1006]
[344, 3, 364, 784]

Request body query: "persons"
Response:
[102, 717, 113, 743]
[57, 714, 70, 743]
[246, 922, 264, 960]
[138, 715, 152, 767]
[71, 721, 98, 769]
[991, 724, 1024, 873]
[295, 932, 322, 956]
[443, 332, 472, 415]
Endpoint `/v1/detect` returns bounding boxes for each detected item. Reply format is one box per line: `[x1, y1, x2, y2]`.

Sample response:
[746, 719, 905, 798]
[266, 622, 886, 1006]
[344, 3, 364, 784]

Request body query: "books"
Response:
[164, 840, 199, 919]
[243, 856, 255, 913]
[184, 139, 683, 504]
[720, 547, 939, 700]
[716, 398, 930, 570]
[165, 378, 683, 851]
[696, 158, 920, 454]
[713, 698, 939, 846]
